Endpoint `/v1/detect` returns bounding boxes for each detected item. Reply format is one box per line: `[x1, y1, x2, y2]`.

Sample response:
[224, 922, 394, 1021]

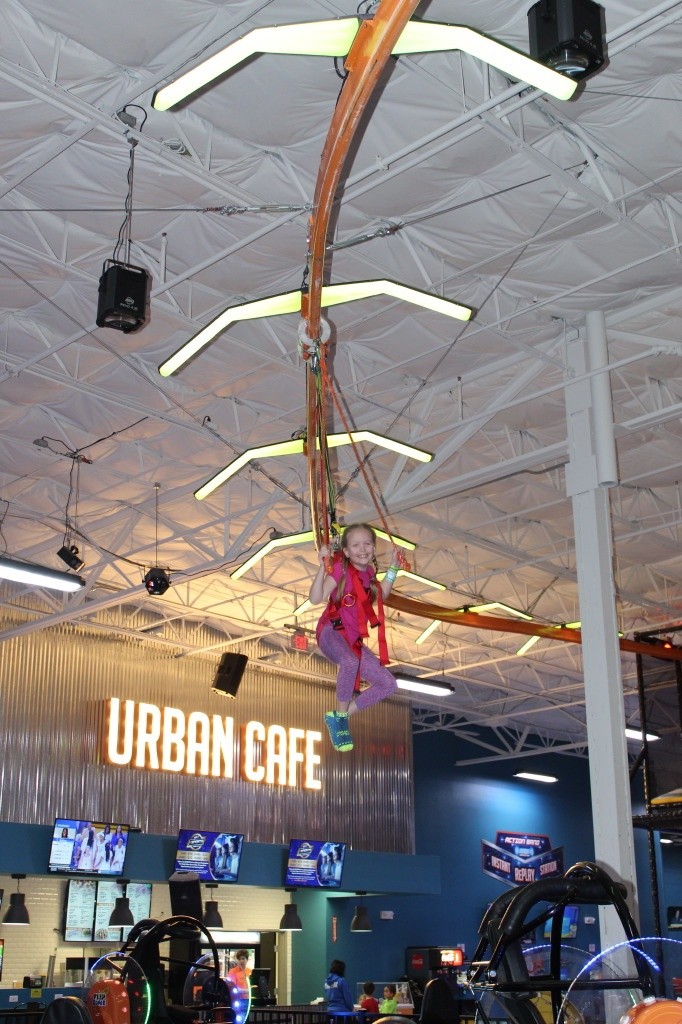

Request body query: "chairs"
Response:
[419, 978, 461, 1024]
[40, 996, 94, 1024]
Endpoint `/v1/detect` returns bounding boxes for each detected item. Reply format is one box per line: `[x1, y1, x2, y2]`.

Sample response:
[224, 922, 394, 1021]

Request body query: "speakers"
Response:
[95, 265, 148, 333]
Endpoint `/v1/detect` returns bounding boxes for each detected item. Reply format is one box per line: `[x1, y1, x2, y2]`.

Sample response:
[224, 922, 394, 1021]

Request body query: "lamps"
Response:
[625, 724, 662, 742]
[109, 879, 134, 927]
[527, 0, 605, 80]
[350, 891, 373, 933]
[512, 768, 559, 783]
[57, 452, 85, 572]
[96, 135, 147, 334]
[210, 642, 248, 700]
[203, 884, 223, 930]
[389, 619, 455, 697]
[280, 888, 302, 932]
[142, 482, 170, 596]
[1, 874, 30, 925]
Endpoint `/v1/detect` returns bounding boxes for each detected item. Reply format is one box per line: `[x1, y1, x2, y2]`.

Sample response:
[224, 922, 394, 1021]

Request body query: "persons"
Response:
[62, 828, 68, 838]
[75, 822, 125, 871]
[193, 955, 210, 998]
[228, 950, 252, 999]
[214, 838, 239, 879]
[324, 960, 354, 1024]
[321, 847, 342, 885]
[360, 983, 379, 1020]
[308, 523, 405, 752]
[379, 985, 397, 1014]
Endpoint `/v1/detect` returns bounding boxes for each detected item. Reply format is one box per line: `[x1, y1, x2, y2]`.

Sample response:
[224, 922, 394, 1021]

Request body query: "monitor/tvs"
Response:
[48, 818, 130, 878]
[287, 838, 347, 887]
[175, 829, 244, 883]
